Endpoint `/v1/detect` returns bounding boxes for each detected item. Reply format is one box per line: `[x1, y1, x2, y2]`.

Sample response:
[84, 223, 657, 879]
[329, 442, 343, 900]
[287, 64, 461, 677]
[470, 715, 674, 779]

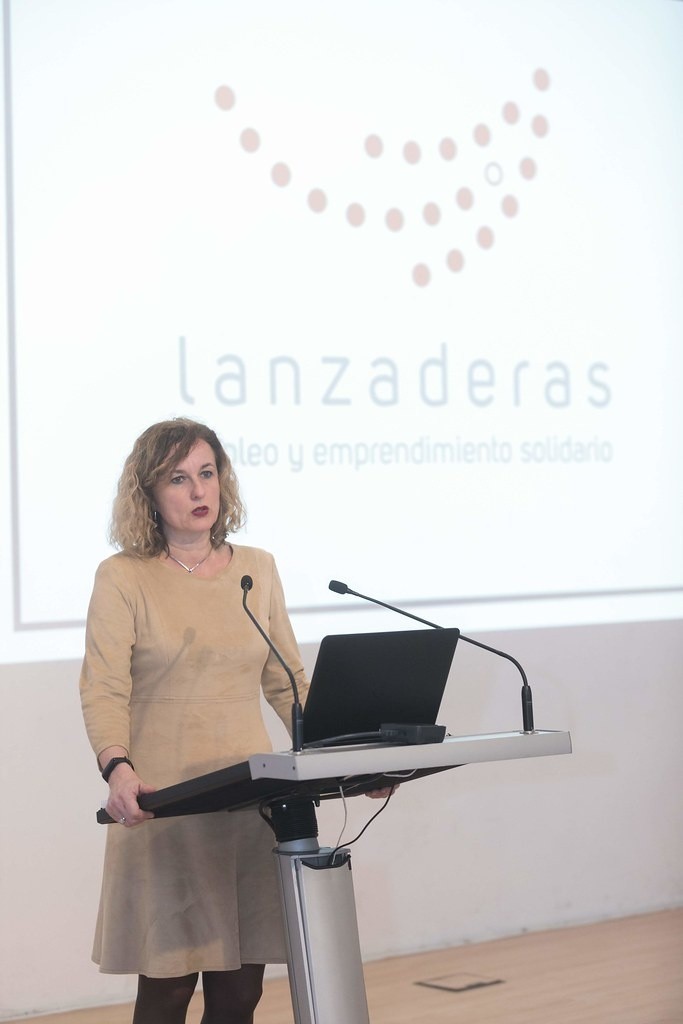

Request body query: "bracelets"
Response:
[102, 756, 134, 783]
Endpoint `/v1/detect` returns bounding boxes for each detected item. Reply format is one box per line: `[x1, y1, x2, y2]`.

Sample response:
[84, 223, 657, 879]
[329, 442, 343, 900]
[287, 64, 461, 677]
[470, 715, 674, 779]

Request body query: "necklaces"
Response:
[163, 545, 214, 574]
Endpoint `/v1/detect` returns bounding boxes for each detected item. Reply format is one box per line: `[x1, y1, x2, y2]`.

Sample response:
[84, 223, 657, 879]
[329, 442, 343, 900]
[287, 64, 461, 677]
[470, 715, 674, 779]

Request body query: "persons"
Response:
[79, 418, 400, 1024]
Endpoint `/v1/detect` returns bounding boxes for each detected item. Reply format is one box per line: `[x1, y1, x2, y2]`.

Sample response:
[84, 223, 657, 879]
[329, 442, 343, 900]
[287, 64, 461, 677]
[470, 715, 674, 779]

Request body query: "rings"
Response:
[118, 817, 127, 825]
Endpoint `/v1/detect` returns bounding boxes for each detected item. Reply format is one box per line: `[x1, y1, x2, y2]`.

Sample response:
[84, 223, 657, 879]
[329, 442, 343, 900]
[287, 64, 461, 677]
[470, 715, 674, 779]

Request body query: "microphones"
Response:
[329, 581, 535, 733]
[241, 574, 305, 752]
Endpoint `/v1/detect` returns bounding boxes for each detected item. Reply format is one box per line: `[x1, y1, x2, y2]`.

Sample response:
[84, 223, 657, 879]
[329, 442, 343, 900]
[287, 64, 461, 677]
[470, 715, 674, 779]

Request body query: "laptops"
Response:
[302, 627, 460, 750]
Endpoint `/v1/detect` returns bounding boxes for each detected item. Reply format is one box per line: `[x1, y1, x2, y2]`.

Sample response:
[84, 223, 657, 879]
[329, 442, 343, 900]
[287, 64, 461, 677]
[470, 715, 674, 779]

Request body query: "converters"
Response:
[378, 722, 446, 744]
